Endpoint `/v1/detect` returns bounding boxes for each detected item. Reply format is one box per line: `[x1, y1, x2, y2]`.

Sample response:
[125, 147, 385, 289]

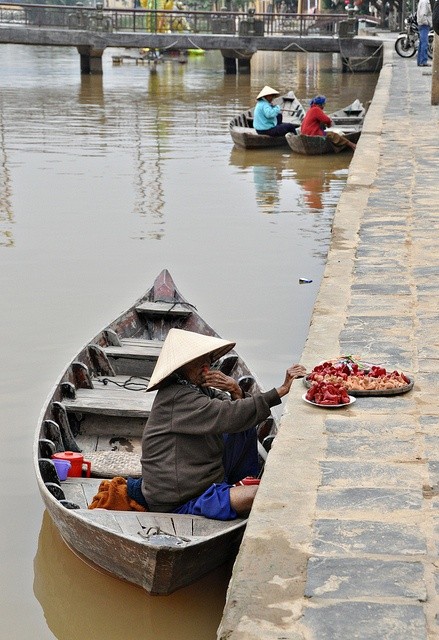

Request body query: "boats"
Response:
[229, 90, 307, 150]
[285, 98, 367, 155]
[32, 268, 279, 596]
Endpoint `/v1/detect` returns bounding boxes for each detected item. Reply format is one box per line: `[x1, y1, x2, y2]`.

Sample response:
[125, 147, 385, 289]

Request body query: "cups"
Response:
[52, 458, 72, 481]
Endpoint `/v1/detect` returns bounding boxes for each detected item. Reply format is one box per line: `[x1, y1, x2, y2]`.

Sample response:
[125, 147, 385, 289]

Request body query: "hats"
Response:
[145, 328, 235, 391]
[256, 86, 280, 100]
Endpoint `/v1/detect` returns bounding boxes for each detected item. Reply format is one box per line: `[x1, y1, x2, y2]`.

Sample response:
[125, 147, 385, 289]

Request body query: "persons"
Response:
[253, 85, 297, 136]
[139, 326, 307, 519]
[415, 0, 433, 67]
[301, 95, 357, 151]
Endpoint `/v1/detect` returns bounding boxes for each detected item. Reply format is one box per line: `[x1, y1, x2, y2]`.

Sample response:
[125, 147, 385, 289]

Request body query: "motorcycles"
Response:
[395, 11, 435, 60]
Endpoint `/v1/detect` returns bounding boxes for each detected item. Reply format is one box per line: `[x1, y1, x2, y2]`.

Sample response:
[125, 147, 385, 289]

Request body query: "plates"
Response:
[303, 391, 356, 407]
